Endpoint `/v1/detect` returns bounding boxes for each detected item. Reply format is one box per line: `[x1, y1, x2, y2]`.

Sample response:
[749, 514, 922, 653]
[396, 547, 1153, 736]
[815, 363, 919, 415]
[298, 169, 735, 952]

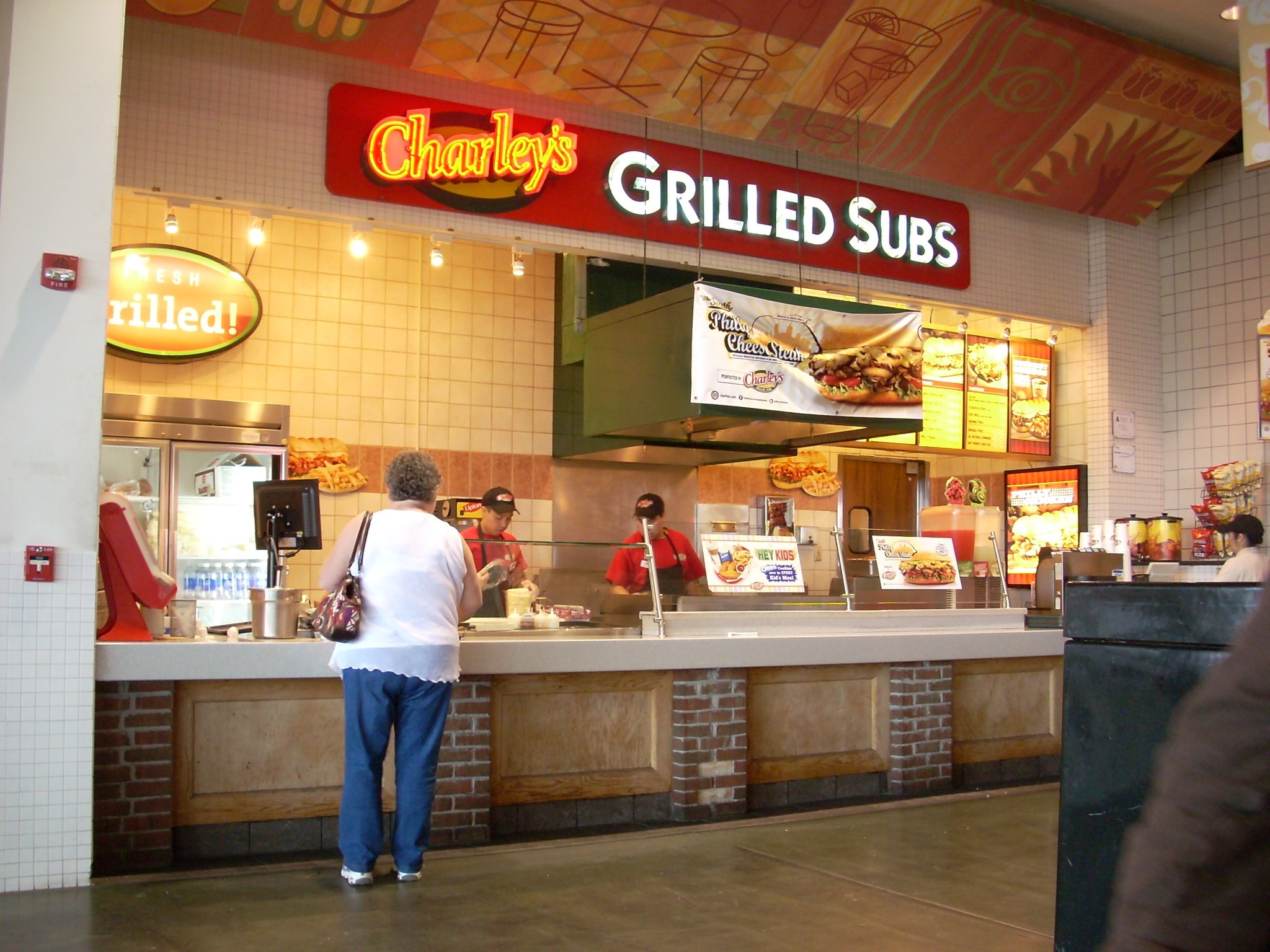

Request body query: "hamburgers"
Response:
[899, 551, 956, 585]
[800, 325, 922, 403]
[1011, 505, 1078, 567]
[921, 336, 1008, 386]
[285, 436, 349, 476]
[1011, 397, 1051, 438]
[769, 451, 828, 489]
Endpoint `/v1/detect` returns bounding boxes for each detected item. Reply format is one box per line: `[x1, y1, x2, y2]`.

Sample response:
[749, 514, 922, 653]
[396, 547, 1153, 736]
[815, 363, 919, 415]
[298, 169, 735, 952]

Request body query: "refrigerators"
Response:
[97, 391, 289, 634]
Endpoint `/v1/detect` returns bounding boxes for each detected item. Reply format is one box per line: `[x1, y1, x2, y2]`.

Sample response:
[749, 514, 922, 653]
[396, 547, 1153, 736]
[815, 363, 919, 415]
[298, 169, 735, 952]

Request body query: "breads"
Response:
[718, 561, 740, 581]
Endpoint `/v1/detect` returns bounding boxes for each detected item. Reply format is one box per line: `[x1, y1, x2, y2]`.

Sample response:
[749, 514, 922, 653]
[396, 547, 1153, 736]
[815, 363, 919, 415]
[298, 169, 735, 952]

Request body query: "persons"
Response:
[1213, 514, 1270, 582]
[604, 492, 706, 596]
[317, 452, 483, 886]
[460, 486, 540, 617]
[1109, 566, 1270, 952]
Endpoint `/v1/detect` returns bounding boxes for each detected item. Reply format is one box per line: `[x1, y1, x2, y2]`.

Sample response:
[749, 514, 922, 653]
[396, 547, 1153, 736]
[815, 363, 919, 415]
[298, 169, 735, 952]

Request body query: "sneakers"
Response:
[340, 862, 374, 885]
[391, 861, 422, 882]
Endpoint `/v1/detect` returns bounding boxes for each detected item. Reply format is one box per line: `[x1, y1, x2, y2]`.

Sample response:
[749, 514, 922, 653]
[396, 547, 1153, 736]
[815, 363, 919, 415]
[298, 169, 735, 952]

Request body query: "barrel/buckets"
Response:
[247, 587, 304, 639]
[1115, 513, 1148, 562]
[1147, 512, 1183, 561]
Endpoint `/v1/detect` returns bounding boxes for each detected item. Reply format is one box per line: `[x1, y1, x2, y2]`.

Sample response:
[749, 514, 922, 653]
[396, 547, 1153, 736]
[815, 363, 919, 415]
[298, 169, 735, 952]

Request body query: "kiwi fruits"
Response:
[967, 479, 986, 504]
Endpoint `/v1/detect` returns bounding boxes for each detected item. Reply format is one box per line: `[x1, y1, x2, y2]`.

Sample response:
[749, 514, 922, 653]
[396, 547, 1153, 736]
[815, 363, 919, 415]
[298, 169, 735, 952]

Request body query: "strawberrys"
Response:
[944, 477, 966, 503]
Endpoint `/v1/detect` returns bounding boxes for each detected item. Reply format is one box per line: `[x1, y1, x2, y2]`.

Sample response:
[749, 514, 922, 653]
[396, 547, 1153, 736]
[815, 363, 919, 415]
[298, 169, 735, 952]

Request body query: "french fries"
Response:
[732, 550, 752, 566]
[306, 460, 368, 490]
[801, 470, 840, 495]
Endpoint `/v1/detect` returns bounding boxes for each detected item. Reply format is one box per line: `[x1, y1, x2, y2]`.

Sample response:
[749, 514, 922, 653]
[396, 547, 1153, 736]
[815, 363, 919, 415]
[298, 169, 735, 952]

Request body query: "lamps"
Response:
[165, 206, 179, 234]
[512, 253, 526, 276]
[430, 244, 444, 267]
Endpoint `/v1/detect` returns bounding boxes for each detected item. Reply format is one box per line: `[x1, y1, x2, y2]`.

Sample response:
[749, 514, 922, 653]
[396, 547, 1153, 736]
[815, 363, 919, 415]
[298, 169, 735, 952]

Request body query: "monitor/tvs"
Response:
[254, 478, 322, 551]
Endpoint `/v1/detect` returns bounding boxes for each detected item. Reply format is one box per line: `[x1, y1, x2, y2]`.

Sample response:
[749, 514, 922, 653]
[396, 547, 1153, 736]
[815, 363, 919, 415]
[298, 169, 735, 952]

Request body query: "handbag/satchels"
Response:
[310, 511, 373, 642]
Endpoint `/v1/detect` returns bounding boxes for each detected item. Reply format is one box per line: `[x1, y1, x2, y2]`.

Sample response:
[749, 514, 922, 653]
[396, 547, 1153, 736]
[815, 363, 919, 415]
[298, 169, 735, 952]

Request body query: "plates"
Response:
[319, 486, 360, 493]
[967, 352, 1006, 385]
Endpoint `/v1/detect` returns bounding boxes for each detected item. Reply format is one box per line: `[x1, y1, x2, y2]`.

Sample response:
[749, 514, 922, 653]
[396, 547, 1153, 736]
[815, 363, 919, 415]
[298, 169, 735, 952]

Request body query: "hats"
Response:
[481, 486, 521, 515]
[1216, 514, 1264, 544]
[632, 493, 664, 521]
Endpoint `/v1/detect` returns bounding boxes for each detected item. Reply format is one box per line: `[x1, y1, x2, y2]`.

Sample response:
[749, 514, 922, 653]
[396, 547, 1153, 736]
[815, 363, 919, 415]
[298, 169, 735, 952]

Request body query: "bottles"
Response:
[478, 558, 514, 589]
[183, 562, 267, 598]
[507, 606, 559, 629]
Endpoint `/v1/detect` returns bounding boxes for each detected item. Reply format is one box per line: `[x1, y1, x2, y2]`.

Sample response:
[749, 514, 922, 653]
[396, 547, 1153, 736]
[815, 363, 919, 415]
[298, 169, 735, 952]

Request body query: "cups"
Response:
[1031, 378, 1048, 398]
[1079, 519, 1132, 582]
[503, 590, 532, 618]
